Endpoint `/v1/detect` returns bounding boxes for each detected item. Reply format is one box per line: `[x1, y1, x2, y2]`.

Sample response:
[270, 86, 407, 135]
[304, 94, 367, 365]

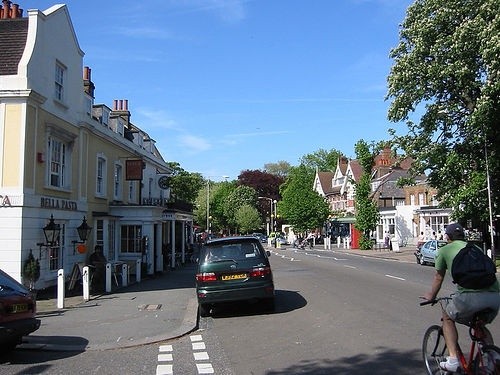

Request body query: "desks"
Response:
[104, 261, 125, 286]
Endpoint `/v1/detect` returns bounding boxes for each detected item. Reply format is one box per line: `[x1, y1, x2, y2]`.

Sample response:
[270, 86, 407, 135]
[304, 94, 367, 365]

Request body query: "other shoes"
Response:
[478, 351, 500, 369]
[439, 356, 461, 373]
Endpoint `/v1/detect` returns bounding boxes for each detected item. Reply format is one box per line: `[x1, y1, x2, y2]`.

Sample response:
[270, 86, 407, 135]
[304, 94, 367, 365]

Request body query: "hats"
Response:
[445, 223, 464, 234]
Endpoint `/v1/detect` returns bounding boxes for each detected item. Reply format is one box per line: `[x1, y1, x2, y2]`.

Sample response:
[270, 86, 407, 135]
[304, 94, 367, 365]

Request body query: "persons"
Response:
[89, 246, 107, 273]
[430, 232, 437, 240]
[384, 231, 390, 245]
[437, 231, 443, 240]
[424, 224, 500, 375]
[418, 232, 424, 240]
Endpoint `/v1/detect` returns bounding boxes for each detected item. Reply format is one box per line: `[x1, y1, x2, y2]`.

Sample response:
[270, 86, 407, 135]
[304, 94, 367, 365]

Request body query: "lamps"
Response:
[71, 215, 94, 245]
[37, 214, 61, 247]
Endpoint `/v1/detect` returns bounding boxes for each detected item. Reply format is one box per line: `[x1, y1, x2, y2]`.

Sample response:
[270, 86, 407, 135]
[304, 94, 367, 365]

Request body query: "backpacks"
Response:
[451, 242, 497, 289]
